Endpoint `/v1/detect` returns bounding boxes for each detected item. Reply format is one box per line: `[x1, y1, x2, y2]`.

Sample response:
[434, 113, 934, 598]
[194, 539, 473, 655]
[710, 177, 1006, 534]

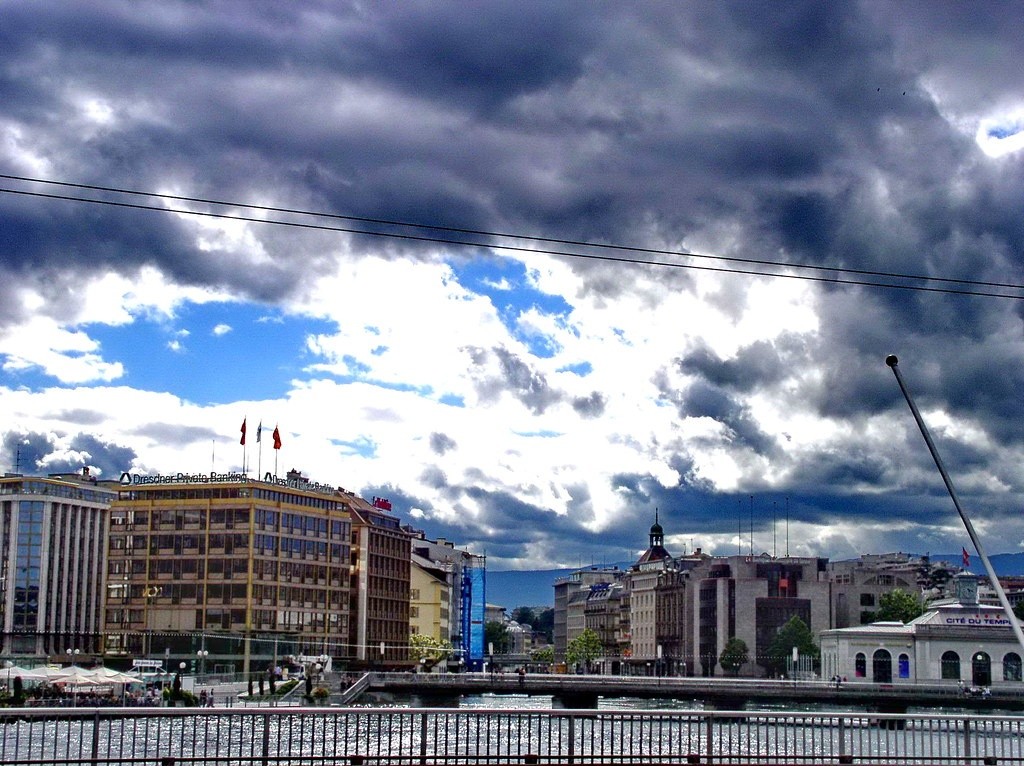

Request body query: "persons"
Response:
[412, 667, 416, 682]
[961, 679, 965, 696]
[518, 668, 525, 682]
[0, 681, 163, 708]
[340, 679, 347, 692]
[208, 688, 214, 696]
[836, 675, 841, 686]
[202, 689, 208, 707]
[957, 679, 962, 698]
[843, 676, 847, 682]
[982, 684, 992, 699]
[347, 677, 356, 688]
[199, 690, 203, 706]
[829, 675, 836, 681]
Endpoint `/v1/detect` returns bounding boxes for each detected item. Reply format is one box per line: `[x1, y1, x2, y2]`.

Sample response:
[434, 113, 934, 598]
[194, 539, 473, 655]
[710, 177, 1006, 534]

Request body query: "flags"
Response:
[239, 419, 247, 445]
[962, 549, 970, 566]
[272, 425, 282, 450]
[256, 424, 261, 442]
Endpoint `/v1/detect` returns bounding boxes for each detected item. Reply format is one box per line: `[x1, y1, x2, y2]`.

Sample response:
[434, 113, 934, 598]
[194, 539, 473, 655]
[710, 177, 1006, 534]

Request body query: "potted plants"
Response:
[160, 671, 185, 707]
[293, 686, 331, 705]
